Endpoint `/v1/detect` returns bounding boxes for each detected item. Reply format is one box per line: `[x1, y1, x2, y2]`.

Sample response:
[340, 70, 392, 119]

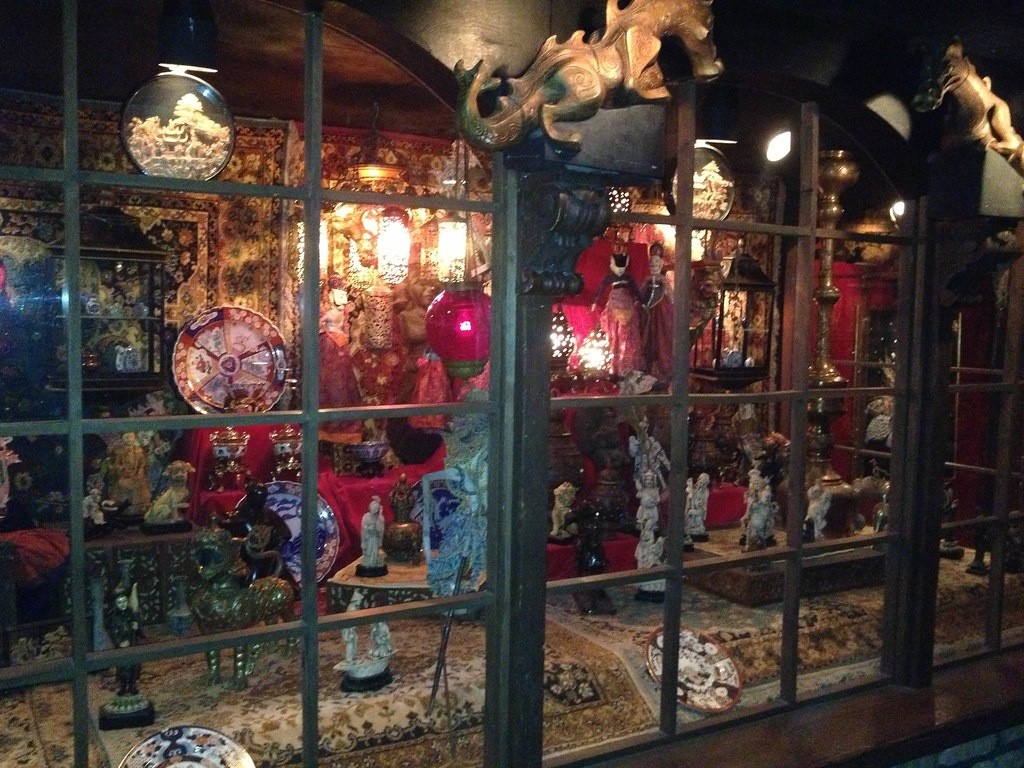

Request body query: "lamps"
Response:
[437, 118, 467, 284]
[426, 117, 490, 389]
[690, 234, 779, 389]
[288, 98, 420, 352]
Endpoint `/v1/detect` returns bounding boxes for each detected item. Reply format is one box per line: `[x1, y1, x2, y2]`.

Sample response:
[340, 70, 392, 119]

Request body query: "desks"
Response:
[1, 522, 197, 652]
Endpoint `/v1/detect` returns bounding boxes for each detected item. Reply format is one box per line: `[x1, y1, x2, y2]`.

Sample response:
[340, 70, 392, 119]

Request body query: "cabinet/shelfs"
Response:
[46, 206, 176, 392]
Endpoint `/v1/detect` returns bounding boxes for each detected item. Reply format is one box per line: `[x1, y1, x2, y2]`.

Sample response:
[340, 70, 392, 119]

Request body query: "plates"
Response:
[406, 478, 463, 550]
[644, 624, 742, 714]
[117, 725, 256, 768]
[234, 480, 341, 589]
[171, 305, 290, 414]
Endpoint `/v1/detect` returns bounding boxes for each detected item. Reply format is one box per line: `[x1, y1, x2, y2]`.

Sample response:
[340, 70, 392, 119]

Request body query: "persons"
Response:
[107, 241, 894, 706]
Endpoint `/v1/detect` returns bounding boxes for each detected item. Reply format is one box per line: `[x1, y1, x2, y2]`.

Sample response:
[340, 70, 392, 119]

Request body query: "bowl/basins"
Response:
[345, 441, 391, 462]
